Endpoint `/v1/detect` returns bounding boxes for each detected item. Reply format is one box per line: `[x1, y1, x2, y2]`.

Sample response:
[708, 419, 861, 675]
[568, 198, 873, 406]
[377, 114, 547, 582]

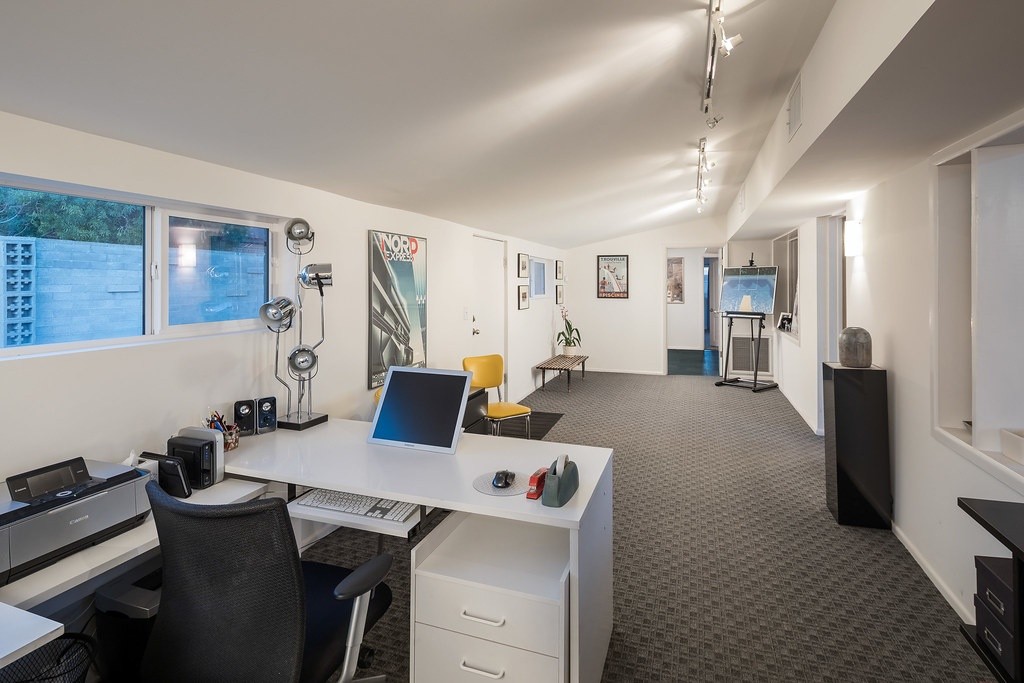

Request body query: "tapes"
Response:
[555, 453, 569, 478]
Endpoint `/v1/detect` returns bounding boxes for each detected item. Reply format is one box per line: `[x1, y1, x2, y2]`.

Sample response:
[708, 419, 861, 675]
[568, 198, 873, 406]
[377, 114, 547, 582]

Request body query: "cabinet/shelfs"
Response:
[957, 497, 1024, 683]
[409, 508, 571, 683]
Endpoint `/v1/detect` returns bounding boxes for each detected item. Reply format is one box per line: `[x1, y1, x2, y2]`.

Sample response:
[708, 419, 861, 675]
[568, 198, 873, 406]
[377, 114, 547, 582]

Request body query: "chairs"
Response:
[462, 354, 533, 439]
[146, 480, 395, 683]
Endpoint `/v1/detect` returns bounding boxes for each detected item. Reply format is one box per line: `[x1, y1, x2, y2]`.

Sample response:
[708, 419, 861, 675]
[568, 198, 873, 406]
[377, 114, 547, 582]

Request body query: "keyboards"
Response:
[297, 488, 420, 522]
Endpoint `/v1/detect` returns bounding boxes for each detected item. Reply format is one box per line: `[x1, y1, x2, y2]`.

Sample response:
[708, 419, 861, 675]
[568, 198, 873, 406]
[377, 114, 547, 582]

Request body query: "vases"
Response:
[563, 345, 576, 358]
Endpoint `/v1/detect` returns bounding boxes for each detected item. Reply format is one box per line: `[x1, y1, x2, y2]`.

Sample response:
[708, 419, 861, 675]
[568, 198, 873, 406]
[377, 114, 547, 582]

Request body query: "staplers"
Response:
[526, 466, 550, 500]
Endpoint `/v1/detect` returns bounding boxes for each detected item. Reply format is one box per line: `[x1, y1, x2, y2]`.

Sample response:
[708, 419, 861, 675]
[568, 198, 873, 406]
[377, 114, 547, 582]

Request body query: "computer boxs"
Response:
[94, 557, 163, 683]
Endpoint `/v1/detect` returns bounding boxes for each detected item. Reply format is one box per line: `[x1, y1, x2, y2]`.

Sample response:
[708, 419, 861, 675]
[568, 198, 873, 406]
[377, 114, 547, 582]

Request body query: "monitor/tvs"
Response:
[367, 366, 473, 455]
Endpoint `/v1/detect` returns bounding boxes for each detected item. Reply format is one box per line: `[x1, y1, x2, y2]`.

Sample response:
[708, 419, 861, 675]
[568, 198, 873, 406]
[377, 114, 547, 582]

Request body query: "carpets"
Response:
[488, 410, 564, 441]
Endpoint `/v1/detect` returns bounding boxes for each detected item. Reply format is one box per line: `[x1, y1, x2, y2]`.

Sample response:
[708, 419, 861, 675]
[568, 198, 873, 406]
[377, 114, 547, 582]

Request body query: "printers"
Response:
[0, 456, 152, 588]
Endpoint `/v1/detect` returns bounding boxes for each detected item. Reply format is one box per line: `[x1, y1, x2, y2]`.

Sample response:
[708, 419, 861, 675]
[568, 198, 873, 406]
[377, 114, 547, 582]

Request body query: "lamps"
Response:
[258, 217, 333, 432]
[697, 149, 719, 214]
[711, 12, 744, 58]
[705, 103, 724, 129]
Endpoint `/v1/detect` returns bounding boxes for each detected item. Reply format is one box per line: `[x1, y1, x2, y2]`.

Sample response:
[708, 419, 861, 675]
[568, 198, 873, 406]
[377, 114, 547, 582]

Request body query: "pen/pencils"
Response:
[200, 405, 237, 432]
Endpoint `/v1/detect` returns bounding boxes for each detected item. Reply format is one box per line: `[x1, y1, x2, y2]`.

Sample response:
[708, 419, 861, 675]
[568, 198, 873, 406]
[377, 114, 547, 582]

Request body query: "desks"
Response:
[0, 475, 270, 683]
[220, 417, 615, 683]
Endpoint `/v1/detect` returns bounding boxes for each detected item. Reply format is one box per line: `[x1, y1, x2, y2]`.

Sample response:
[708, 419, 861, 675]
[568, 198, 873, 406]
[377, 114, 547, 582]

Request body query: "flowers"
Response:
[555, 306, 583, 348]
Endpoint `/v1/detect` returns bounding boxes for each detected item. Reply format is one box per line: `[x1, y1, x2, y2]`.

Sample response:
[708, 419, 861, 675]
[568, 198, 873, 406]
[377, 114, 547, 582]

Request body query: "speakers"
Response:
[234, 400, 256, 435]
[255, 396, 278, 435]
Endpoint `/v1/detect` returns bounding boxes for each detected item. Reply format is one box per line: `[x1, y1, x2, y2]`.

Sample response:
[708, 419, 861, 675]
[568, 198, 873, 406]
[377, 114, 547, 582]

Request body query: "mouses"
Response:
[492, 470, 516, 489]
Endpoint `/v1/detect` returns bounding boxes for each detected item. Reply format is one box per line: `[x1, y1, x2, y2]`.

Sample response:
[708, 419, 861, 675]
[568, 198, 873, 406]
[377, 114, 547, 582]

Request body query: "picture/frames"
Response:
[556, 285, 564, 304]
[597, 255, 629, 299]
[518, 253, 529, 278]
[518, 285, 530, 311]
[555, 260, 564, 280]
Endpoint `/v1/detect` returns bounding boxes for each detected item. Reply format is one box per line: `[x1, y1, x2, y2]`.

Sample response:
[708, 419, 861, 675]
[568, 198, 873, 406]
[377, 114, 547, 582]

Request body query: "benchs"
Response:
[535, 354, 590, 390]
